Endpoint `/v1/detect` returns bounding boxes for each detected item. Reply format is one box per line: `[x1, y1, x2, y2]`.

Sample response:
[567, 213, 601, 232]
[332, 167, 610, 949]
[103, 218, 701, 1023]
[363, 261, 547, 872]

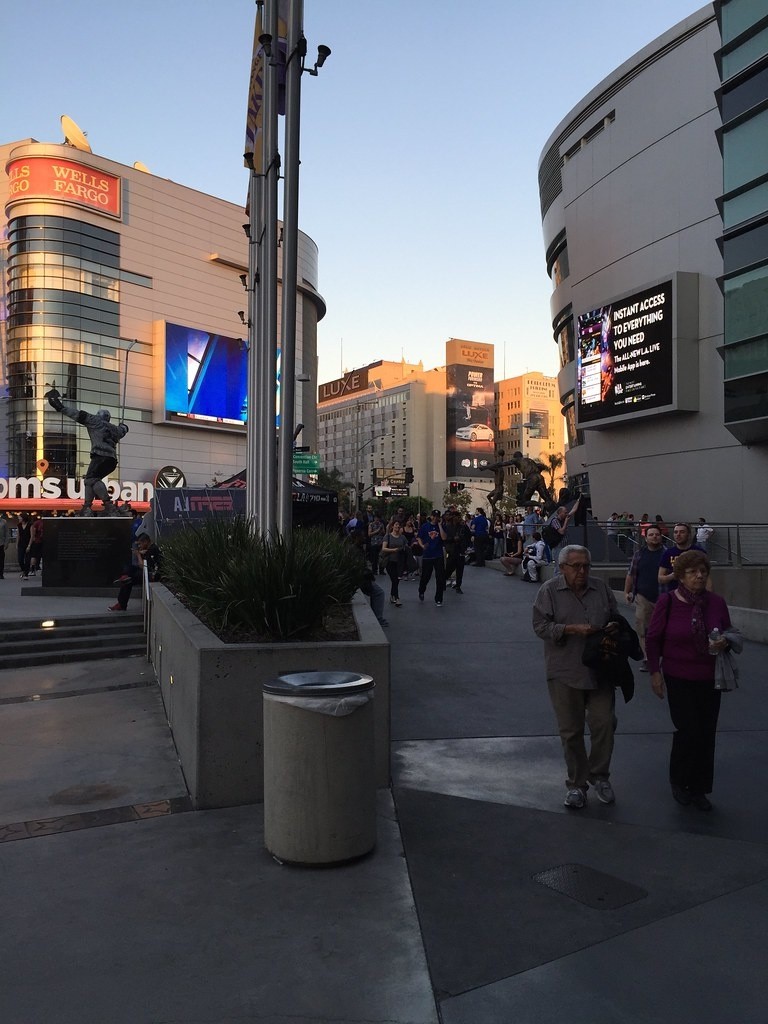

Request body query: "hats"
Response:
[451, 511, 459, 517]
[431, 510, 441, 516]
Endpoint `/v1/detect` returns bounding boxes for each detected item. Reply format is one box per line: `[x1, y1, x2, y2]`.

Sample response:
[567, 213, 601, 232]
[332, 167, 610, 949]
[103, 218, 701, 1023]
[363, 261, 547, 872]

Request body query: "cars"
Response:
[455, 423, 494, 441]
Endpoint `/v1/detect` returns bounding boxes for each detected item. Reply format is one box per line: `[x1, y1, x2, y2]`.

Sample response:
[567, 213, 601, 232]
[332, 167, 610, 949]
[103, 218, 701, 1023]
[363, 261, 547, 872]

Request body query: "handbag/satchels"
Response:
[378, 549, 390, 567]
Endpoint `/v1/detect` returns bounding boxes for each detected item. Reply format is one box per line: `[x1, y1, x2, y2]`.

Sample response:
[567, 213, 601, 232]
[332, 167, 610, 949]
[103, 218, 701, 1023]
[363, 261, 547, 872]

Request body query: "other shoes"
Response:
[678, 792, 711, 810]
[411, 578, 415, 582]
[0, 566, 43, 580]
[373, 571, 378, 575]
[472, 563, 485, 567]
[404, 576, 408, 581]
[418, 589, 424, 602]
[455, 585, 464, 594]
[435, 600, 442, 607]
[380, 571, 386, 575]
[379, 619, 390, 627]
[395, 600, 401, 606]
[639, 660, 649, 671]
[503, 572, 515, 576]
[529, 580, 538, 583]
[390, 597, 396, 603]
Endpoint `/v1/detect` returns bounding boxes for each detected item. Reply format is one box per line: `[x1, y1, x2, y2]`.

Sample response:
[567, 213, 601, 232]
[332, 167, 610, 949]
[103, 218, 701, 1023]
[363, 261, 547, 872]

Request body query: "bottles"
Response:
[626, 593, 633, 606]
[708, 627, 721, 656]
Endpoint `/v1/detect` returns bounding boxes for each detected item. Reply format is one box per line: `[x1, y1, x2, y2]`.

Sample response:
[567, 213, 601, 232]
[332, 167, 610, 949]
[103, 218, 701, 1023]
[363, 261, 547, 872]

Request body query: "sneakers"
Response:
[596, 778, 615, 804]
[564, 788, 586, 807]
[112, 577, 134, 586]
[107, 601, 128, 612]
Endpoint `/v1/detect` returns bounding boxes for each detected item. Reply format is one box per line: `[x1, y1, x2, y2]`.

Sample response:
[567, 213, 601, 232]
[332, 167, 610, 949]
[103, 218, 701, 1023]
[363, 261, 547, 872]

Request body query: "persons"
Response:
[463, 404, 476, 420]
[15, 514, 43, 580]
[625, 525, 669, 671]
[49, 396, 128, 516]
[478, 451, 556, 510]
[659, 523, 713, 593]
[0, 518, 9, 579]
[343, 505, 540, 626]
[108, 533, 164, 611]
[696, 518, 714, 549]
[550, 493, 582, 577]
[607, 512, 669, 556]
[532, 544, 621, 808]
[129, 509, 142, 560]
[487, 456, 504, 516]
[645, 549, 732, 811]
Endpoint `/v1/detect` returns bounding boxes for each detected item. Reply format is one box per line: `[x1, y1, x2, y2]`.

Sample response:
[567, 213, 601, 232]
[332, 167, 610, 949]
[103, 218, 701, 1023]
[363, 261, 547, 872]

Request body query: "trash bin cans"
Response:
[259, 672, 380, 869]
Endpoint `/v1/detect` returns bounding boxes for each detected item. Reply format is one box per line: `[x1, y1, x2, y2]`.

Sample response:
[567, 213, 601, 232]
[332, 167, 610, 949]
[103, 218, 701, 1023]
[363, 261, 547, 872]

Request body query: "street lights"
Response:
[355, 433, 395, 511]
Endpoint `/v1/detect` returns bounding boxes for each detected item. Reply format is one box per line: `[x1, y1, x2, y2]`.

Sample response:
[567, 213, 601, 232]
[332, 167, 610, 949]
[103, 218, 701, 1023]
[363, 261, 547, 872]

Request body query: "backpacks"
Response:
[542, 519, 565, 548]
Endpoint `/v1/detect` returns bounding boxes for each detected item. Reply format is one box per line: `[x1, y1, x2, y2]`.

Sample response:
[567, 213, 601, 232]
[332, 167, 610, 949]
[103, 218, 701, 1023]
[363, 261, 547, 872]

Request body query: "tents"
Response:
[211, 469, 338, 533]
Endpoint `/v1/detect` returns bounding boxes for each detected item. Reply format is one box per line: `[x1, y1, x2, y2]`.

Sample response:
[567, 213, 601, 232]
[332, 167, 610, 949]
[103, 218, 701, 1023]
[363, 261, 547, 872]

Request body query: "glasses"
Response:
[561, 563, 593, 571]
[684, 567, 707, 576]
[675, 523, 687, 527]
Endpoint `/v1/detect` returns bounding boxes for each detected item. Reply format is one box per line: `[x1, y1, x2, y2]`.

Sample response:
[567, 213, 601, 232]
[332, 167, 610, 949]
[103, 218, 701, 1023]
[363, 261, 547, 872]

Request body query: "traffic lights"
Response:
[406, 468, 414, 484]
[450, 482, 458, 494]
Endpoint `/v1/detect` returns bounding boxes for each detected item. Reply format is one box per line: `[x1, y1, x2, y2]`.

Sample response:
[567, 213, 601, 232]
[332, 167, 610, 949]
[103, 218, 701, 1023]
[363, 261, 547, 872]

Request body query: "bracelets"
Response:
[577, 499, 580, 502]
